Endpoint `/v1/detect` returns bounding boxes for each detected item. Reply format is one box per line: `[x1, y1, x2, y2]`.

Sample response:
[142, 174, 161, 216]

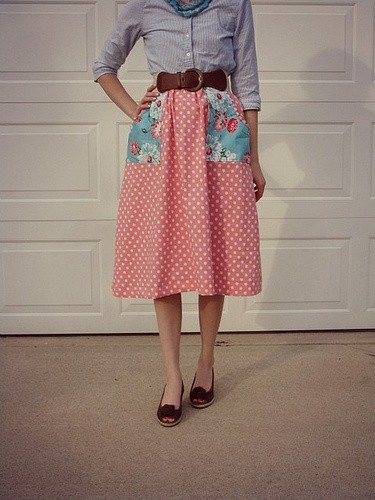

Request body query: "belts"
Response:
[153, 70, 227, 94]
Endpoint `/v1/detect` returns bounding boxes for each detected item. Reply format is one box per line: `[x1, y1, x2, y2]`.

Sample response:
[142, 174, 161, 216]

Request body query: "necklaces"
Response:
[166, 0, 206, 10]
[167, 0, 213, 17]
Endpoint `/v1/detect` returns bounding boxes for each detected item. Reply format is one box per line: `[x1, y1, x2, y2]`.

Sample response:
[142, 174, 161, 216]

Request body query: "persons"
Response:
[91, 0, 267, 429]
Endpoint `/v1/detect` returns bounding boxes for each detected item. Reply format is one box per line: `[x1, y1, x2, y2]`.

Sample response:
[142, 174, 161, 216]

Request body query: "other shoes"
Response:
[190, 367, 216, 409]
[157, 379, 184, 427]
